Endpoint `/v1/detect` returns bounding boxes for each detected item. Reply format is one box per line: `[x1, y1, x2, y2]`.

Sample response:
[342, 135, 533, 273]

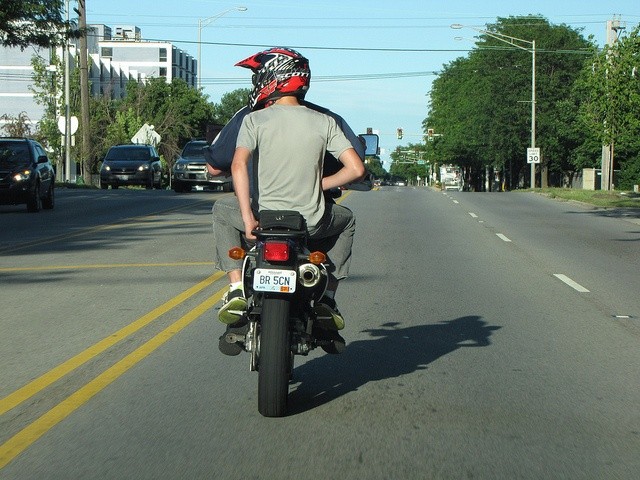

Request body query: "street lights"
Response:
[197, 6, 248, 90]
[450, 24, 537, 189]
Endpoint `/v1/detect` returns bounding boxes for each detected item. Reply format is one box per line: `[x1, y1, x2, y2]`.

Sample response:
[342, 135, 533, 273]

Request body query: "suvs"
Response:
[100, 143, 164, 190]
[172, 139, 233, 193]
[0, 136, 55, 213]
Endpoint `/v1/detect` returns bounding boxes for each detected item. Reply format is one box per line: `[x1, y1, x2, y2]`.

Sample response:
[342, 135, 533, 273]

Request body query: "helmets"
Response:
[233, 47, 310, 112]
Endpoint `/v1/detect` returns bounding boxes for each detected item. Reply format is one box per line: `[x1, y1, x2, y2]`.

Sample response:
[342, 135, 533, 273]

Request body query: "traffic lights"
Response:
[396, 128, 403, 139]
[367, 128, 372, 134]
[428, 129, 434, 142]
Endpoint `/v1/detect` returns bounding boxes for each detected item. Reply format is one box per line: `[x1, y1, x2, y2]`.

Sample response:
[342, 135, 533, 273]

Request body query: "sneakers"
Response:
[219, 314, 251, 356]
[312, 324, 345, 354]
[217, 289, 248, 325]
[313, 294, 344, 330]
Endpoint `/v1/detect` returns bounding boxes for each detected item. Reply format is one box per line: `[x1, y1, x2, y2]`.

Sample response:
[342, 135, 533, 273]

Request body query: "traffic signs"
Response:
[526, 147, 540, 164]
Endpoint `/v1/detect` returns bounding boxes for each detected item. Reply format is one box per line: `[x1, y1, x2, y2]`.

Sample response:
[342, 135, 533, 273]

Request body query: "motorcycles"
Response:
[226, 133, 379, 417]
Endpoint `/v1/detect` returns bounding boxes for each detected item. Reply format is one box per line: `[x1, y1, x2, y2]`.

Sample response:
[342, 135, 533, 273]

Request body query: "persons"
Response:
[210, 45, 356, 331]
[201, 99, 366, 356]
